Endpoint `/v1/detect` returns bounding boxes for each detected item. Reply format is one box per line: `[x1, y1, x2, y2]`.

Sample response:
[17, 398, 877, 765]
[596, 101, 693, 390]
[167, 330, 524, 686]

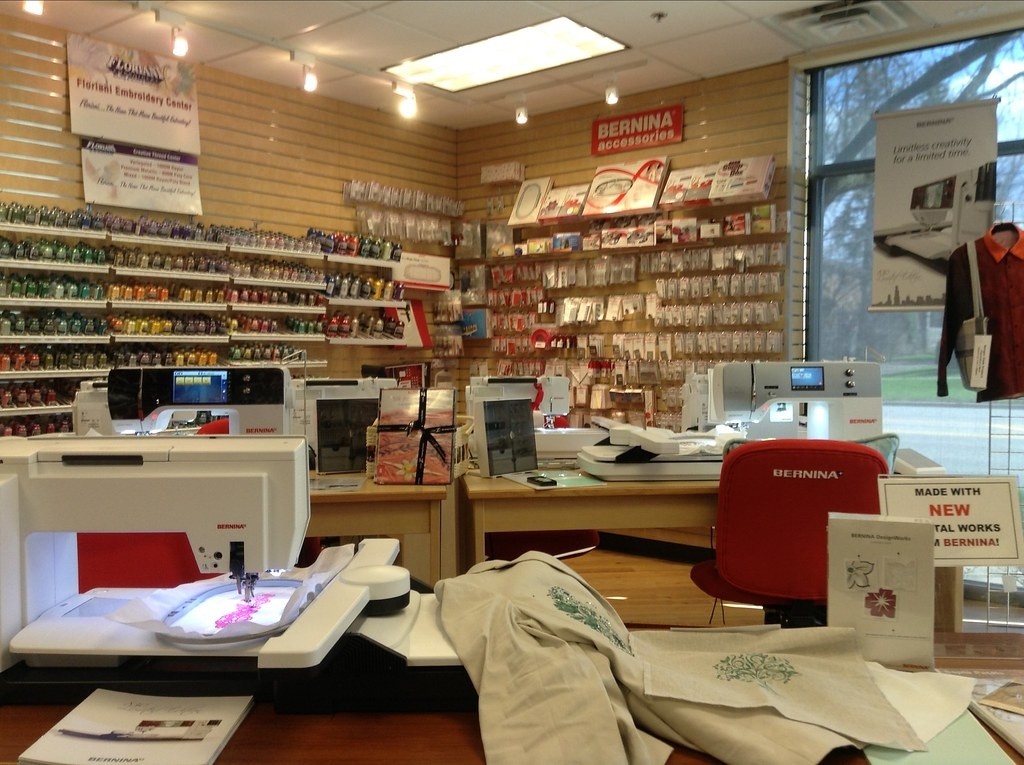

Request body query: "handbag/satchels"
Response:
[953, 240, 992, 392]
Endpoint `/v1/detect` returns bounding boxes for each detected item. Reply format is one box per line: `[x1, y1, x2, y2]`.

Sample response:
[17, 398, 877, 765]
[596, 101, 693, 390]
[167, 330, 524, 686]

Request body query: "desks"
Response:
[304, 467, 447, 590]
[465, 463, 719, 563]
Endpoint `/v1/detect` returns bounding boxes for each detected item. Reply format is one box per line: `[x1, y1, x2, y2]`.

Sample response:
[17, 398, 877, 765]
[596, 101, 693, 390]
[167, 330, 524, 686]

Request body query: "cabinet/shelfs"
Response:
[218, 242, 328, 368]
[326, 253, 410, 345]
[0, 221, 112, 437]
[107, 233, 230, 368]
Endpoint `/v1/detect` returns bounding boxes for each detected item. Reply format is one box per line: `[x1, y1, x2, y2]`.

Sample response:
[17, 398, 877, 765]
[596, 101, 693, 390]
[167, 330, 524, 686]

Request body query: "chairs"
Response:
[689, 439, 890, 631]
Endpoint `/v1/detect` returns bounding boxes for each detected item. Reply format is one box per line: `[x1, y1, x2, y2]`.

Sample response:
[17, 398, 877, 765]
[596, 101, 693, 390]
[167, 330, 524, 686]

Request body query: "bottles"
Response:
[324, 230, 404, 340]
[224, 222, 325, 362]
[106, 211, 227, 368]
[0, 201, 107, 437]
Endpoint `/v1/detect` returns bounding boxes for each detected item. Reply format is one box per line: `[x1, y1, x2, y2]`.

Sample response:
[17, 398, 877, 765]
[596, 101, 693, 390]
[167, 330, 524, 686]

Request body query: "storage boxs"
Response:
[452, 148, 792, 263]
[380, 296, 433, 351]
[389, 250, 451, 291]
[373, 387, 458, 485]
[458, 263, 493, 306]
[459, 308, 494, 340]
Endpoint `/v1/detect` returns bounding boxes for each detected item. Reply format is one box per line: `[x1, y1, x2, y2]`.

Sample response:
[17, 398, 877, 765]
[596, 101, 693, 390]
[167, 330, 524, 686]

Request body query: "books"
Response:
[19, 688, 256, 765]
